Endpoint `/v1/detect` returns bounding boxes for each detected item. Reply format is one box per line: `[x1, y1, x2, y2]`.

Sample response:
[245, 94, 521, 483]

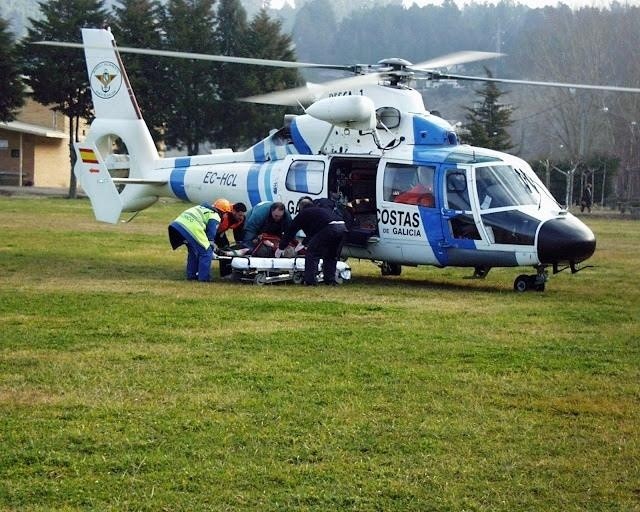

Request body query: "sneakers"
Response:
[186, 277, 216, 282]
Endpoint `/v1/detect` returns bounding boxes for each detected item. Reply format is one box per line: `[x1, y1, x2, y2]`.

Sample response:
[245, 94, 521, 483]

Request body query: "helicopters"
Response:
[31, 28, 640, 292]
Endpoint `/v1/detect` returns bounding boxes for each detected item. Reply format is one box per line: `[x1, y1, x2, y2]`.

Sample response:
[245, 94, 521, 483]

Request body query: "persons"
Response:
[275, 198, 348, 286]
[298, 196, 354, 261]
[214, 234, 308, 257]
[235, 200, 296, 245]
[582, 183, 593, 212]
[211, 202, 245, 279]
[168, 198, 232, 282]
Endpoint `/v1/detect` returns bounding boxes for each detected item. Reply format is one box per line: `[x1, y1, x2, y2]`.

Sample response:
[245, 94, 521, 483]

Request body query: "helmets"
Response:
[214, 199, 232, 213]
